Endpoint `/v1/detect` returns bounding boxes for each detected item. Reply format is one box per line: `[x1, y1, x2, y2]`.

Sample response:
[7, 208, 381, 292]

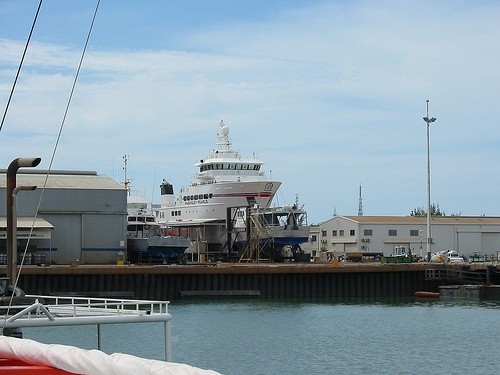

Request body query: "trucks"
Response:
[448, 249, 463, 263]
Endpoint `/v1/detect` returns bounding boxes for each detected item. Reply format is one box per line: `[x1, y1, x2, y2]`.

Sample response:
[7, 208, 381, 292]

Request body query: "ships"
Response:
[150, 120, 282, 244]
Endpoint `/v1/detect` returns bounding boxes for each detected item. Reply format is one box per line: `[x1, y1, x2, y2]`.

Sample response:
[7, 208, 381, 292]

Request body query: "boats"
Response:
[128, 186, 191, 266]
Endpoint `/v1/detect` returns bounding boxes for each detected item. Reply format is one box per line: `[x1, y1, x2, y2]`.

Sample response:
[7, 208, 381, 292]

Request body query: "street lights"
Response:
[423, 99, 437, 263]
